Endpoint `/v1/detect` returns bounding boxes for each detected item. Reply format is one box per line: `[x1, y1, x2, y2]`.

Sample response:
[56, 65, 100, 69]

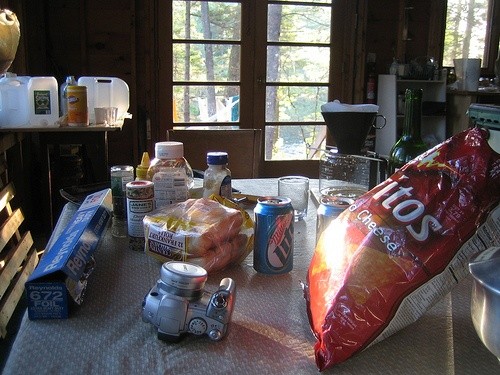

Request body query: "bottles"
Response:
[62, 75, 78, 114]
[135, 151, 151, 181]
[204, 151, 231, 200]
[147, 141, 194, 209]
[389, 87, 429, 177]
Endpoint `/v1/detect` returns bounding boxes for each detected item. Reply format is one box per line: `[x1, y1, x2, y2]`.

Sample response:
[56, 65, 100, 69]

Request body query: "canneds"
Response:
[252, 196, 293, 274]
[66, 86, 90, 127]
[315, 189, 370, 249]
[125, 181, 156, 251]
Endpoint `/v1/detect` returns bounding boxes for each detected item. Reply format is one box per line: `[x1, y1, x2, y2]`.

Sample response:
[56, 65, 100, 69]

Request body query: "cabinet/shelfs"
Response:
[375, 71, 447, 156]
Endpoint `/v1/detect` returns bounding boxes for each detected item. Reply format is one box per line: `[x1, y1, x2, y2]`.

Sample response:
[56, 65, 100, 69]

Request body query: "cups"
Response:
[94, 107, 118, 125]
[321, 111, 386, 156]
[278, 175, 309, 223]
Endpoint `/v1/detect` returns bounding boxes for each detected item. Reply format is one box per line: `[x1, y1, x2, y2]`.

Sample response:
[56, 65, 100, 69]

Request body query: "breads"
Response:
[144, 197, 248, 275]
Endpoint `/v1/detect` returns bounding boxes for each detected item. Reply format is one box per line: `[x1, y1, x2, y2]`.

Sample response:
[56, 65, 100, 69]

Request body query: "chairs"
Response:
[0, 173, 39, 344]
[163, 127, 261, 179]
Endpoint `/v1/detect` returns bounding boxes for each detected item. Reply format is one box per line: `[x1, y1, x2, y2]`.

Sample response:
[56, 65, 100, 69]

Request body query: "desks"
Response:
[0, 179, 500, 375]
[0, 117, 122, 234]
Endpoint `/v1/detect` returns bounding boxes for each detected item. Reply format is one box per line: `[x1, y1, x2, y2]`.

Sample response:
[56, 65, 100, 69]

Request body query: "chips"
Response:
[312, 237, 417, 307]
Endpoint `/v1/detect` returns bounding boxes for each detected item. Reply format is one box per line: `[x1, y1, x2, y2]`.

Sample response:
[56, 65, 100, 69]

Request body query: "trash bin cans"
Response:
[453, 58, 482, 92]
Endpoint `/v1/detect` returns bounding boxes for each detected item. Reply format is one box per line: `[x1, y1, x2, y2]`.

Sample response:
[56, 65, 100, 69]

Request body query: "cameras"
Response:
[140, 261, 235, 344]
